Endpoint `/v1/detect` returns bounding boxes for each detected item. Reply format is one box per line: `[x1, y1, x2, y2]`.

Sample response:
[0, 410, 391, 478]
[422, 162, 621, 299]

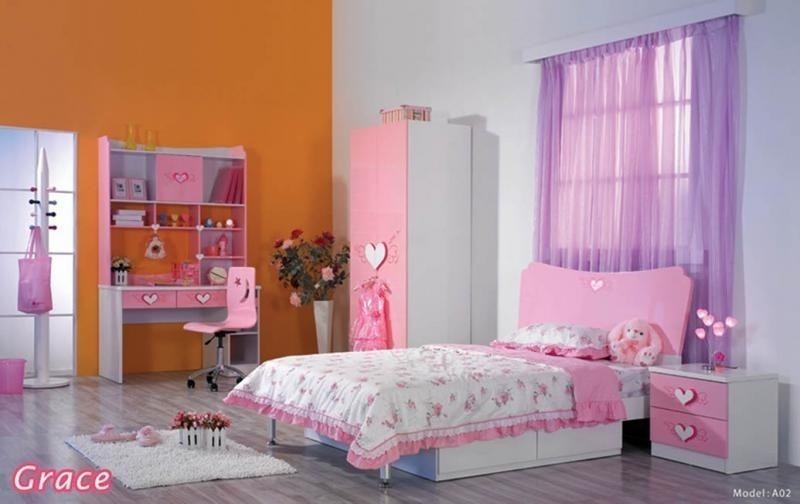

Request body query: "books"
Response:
[113, 209, 146, 225]
[209, 168, 245, 204]
[111, 175, 146, 200]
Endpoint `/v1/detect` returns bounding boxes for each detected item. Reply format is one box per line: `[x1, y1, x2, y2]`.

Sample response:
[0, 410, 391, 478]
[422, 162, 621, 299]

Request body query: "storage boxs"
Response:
[380, 105, 432, 125]
[303, 419, 623, 481]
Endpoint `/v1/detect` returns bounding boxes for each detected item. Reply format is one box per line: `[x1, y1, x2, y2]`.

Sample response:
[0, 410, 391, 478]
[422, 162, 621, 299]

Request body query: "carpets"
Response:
[63, 429, 297, 490]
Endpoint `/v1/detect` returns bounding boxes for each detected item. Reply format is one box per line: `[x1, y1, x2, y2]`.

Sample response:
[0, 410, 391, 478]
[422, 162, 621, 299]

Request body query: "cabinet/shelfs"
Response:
[349, 118, 471, 351]
[98, 135, 261, 384]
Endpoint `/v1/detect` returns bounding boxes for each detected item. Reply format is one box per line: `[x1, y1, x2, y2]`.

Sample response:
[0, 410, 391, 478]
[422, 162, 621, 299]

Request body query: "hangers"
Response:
[23, 148, 70, 390]
[352, 270, 387, 291]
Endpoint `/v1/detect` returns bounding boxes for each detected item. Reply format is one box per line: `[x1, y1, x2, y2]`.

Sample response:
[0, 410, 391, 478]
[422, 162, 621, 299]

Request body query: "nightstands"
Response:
[648, 363, 778, 474]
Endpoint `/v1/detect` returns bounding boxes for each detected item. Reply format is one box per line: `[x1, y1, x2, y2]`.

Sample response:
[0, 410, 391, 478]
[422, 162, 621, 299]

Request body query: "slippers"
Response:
[91, 423, 136, 443]
[136, 425, 163, 447]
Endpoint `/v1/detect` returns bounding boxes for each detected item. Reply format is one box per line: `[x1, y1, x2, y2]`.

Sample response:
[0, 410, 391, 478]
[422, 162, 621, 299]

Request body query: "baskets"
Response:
[380, 102, 433, 129]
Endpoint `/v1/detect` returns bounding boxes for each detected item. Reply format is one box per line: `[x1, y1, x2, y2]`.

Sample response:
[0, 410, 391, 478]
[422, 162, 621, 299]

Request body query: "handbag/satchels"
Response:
[17, 226, 54, 314]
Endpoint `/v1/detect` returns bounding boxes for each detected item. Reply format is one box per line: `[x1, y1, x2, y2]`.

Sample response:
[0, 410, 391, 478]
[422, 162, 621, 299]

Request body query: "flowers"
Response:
[713, 352, 725, 366]
[271, 229, 350, 307]
[169, 409, 204, 430]
[200, 411, 231, 430]
[110, 255, 135, 272]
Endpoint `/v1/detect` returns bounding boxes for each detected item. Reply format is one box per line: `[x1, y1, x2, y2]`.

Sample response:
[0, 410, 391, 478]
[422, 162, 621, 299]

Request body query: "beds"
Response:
[269, 261, 694, 494]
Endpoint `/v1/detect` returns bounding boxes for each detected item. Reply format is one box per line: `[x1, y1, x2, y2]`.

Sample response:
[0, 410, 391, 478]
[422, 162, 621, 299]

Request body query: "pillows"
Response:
[489, 320, 610, 359]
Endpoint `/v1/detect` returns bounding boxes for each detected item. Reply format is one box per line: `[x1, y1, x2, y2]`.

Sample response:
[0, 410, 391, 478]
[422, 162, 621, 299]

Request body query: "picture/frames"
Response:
[129, 177, 147, 200]
[111, 176, 129, 199]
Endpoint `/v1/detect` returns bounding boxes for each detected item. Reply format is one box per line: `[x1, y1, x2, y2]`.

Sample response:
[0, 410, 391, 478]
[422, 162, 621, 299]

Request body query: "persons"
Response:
[607, 318, 673, 367]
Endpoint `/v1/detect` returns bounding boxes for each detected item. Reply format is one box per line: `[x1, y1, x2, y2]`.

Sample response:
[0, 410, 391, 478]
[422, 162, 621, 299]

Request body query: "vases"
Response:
[715, 367, 726, 375]
[179, 426, 204, 449]
[113, 270, 128, 285]
[206, 427, 225, 449]
[312, 300, 334, 354]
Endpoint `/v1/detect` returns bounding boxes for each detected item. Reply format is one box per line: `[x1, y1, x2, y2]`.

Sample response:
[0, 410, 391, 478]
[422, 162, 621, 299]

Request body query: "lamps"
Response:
[695, 308, 738, 370]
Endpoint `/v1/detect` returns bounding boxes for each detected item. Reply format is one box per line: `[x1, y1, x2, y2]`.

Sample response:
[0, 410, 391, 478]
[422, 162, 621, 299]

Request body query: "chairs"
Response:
[183, 266, 257, 392]
